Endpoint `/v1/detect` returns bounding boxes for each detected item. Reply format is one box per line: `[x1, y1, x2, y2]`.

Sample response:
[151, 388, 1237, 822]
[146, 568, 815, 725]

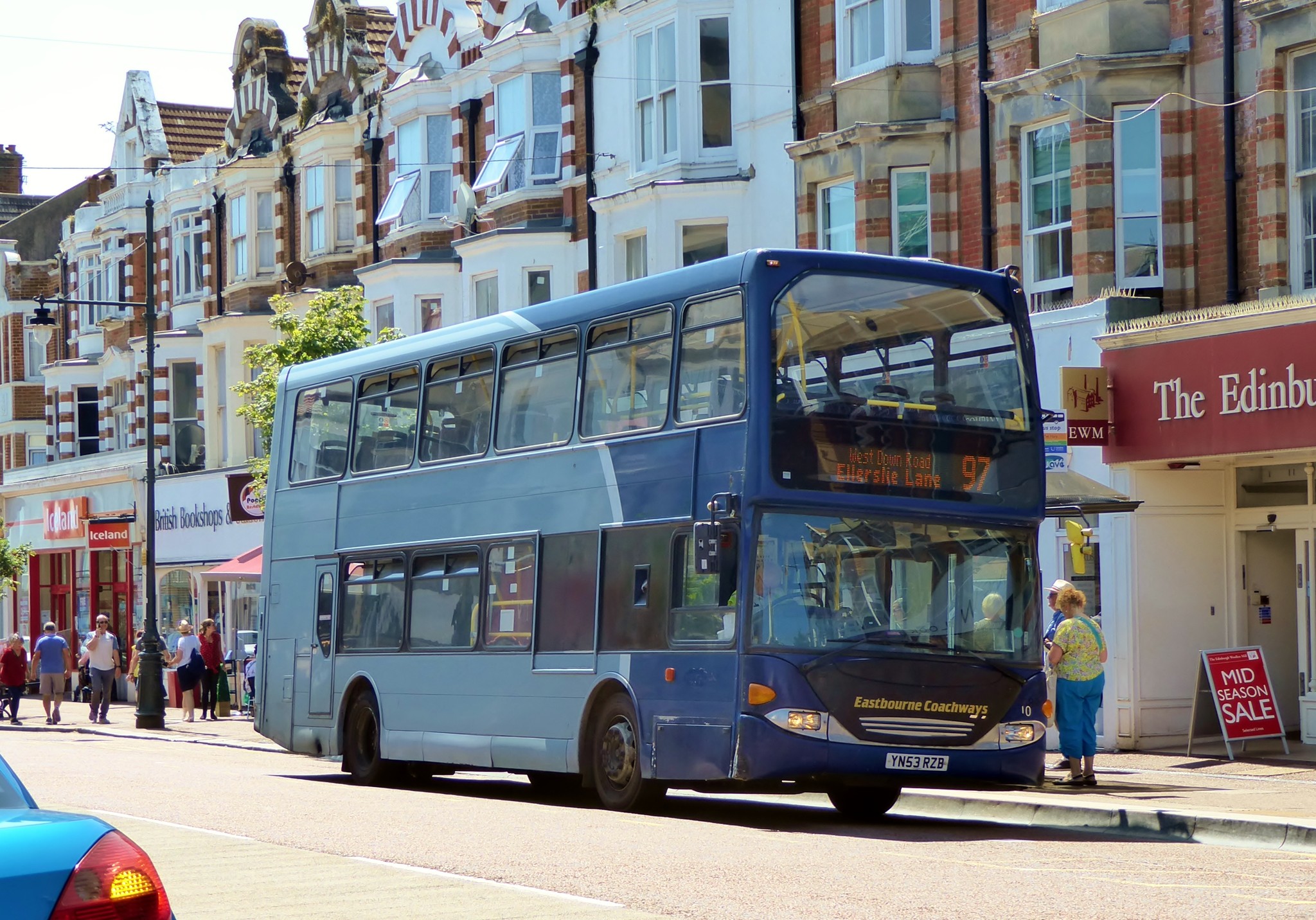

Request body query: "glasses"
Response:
[1049, 593, 1058, 597]
[100, 621, 108, 624]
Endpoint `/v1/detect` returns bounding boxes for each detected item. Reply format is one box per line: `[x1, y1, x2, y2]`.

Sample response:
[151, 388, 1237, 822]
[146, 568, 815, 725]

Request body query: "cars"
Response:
[0, 753, 179, 920]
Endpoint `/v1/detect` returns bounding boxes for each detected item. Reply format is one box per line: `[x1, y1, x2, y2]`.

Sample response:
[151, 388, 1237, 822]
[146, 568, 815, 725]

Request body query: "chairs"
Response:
[315, 383, 958, 480]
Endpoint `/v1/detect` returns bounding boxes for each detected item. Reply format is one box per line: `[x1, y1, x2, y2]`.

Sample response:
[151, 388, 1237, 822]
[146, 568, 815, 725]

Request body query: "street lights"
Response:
[24, 190, 167, 730]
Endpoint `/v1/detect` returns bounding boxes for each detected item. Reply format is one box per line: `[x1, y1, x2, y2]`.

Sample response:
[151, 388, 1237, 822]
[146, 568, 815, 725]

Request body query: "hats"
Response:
[175, 619, 193, 633]
[1044, 579, 1075, 593]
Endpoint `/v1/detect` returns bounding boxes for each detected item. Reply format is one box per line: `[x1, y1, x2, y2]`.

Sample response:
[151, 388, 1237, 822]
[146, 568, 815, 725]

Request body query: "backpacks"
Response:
[184, 635, 205, 675]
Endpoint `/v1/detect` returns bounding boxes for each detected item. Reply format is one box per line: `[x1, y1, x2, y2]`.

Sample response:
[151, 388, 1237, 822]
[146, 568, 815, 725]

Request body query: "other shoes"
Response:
[211, 710, 217, 719]
[89, 704, 98, 721]
[1044, 759, 1082, 771]
[200, 710, 207, 719]
[46, 718, 52, 724]
[99, 717, 110, 724]
[10, 718, 19, 725]
[52, 707, 60, 724]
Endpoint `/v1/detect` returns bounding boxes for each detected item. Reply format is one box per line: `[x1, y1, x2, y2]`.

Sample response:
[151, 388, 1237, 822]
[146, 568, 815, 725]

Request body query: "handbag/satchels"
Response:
[244, 678, 251, 693]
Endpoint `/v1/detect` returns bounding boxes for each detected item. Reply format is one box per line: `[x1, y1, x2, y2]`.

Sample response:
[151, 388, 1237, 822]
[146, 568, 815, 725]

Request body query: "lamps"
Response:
[1168, 462, 1200, 469]
[1255, 515, 1278, 532]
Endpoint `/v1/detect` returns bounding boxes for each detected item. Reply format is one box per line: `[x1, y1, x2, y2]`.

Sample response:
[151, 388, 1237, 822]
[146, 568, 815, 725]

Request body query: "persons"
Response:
[890, 598, 903, 630]
[125, 619, 226, 722]
[727, 561, 798, 643]
[0, 633, 30, 725]
[971, 593, 1006, 650]
[30, 621, 122, 724]
[1044, 579, 1108, 785]
[86, 614, 121, 724]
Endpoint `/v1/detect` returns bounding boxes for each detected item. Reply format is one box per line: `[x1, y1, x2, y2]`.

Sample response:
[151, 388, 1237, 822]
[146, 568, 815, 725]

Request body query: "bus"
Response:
[253, 246, 1101, 817]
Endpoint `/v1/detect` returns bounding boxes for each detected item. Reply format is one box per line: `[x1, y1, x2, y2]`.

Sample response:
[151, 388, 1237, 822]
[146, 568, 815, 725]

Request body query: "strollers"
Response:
[71, 652, 103, 704]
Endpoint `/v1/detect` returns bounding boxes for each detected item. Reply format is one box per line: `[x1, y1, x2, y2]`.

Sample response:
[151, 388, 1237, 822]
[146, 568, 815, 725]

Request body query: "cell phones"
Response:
[96, 624, 100, 628]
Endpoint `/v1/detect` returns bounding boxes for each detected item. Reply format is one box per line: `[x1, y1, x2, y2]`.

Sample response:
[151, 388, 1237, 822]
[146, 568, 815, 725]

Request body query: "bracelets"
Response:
[116, 665, 121, 667]
[95, 635, 99, 638]
[129, 673, 134, 675]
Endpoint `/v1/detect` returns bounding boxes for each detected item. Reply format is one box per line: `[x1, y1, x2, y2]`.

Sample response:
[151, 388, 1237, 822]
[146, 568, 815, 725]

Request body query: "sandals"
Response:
[1082, 773, 1097, 785]
[1052, 774, 1084, 786]
[183, 716, 194, 722]
